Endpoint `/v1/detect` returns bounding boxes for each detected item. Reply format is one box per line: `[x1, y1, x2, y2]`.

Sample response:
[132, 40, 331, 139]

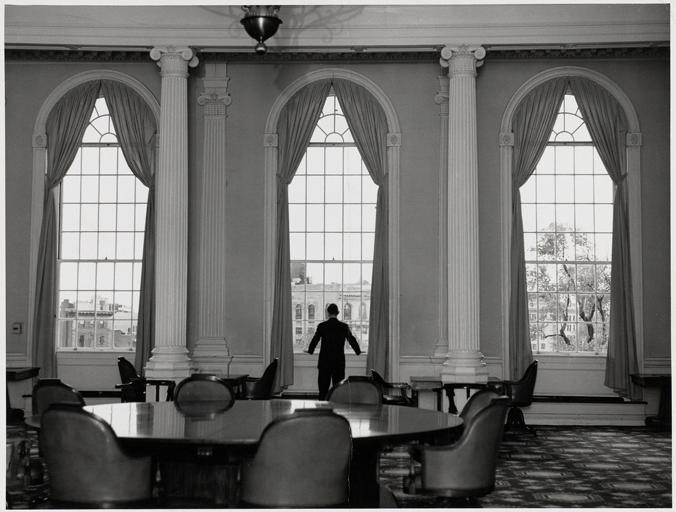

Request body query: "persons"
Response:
[304, 304, 365, 401]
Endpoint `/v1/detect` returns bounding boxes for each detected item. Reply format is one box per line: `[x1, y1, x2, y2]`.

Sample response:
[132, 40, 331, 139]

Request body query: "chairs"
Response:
[370, 368, 416, 407]
[235, 357, 279, 400]
[490, 360, 538, 435]
[236, 413, 353, 509]
[117, 356, 147, 402]
[459, 387, 504, 421]
[31, 378, 86, 414]
[326, 375, 383, 406]
[412, 394, 509, 508]
[39, 403, 153, 509]
[173, 374, 233, 401]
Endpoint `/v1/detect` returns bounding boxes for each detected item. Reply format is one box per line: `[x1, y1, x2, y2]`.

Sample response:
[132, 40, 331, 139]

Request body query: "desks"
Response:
[410, 383, 487, 414]
[80, 398, 465, 508]
[141, 374, 250, 402]
[630, 374, 671, 432]
[7, 366, 41, 422]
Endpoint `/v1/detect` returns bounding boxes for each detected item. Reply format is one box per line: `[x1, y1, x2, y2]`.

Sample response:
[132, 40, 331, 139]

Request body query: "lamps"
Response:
[240, 6, 283, 55]
